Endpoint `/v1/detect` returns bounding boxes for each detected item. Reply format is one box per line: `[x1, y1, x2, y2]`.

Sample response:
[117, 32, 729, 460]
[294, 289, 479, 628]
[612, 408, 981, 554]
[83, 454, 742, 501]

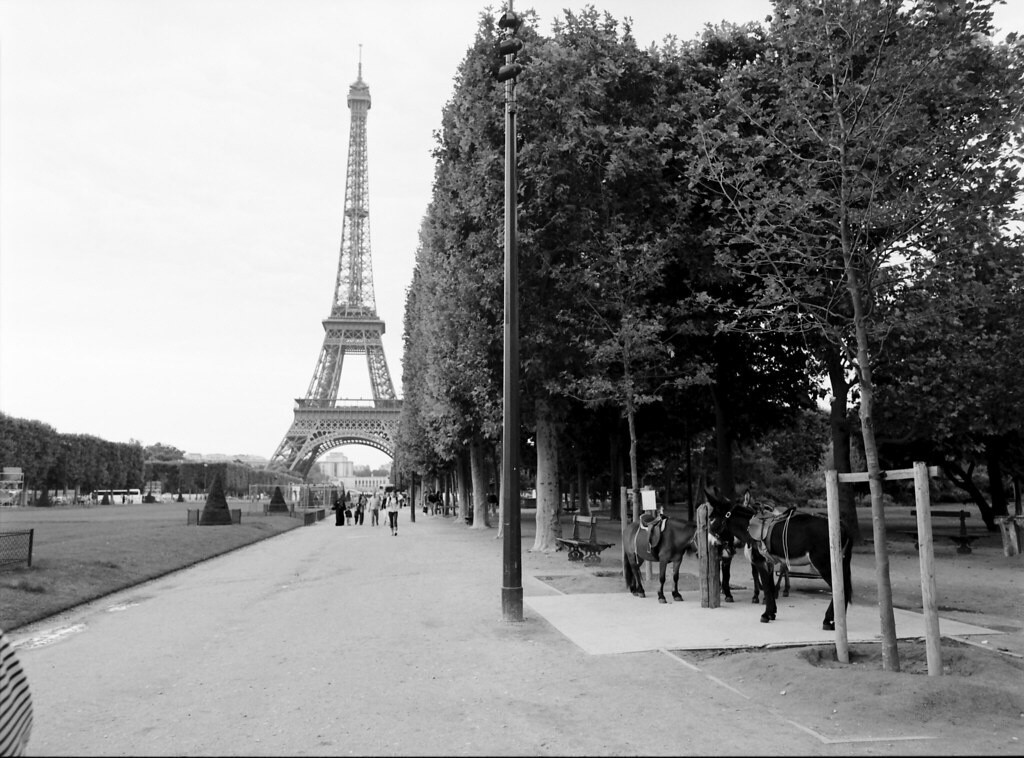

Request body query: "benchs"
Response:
[436, 506, 456, 516]
[555, 514, 616, 566]
[897, 509, 990, 554]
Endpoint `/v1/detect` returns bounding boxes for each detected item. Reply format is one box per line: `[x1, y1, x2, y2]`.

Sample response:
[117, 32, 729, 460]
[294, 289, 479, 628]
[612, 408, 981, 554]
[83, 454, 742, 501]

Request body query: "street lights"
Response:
[495, 11, 524, 623]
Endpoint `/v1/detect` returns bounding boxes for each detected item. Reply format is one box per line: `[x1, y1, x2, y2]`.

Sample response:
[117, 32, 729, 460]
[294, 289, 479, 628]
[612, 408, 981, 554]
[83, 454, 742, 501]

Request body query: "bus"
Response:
[90, 489, 140, 500]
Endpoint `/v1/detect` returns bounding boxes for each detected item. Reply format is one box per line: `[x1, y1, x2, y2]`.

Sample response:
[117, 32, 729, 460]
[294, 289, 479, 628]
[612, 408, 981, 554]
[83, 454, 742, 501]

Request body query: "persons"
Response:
[332, 490, 441, 526]
[488, 491, 499, 517]
[385, 489, 403, 536]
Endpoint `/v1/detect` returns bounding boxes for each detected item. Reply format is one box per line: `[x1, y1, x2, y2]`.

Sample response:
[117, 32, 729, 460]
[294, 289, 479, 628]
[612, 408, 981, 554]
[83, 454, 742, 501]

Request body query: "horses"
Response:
[623, 485, 856, 630]
